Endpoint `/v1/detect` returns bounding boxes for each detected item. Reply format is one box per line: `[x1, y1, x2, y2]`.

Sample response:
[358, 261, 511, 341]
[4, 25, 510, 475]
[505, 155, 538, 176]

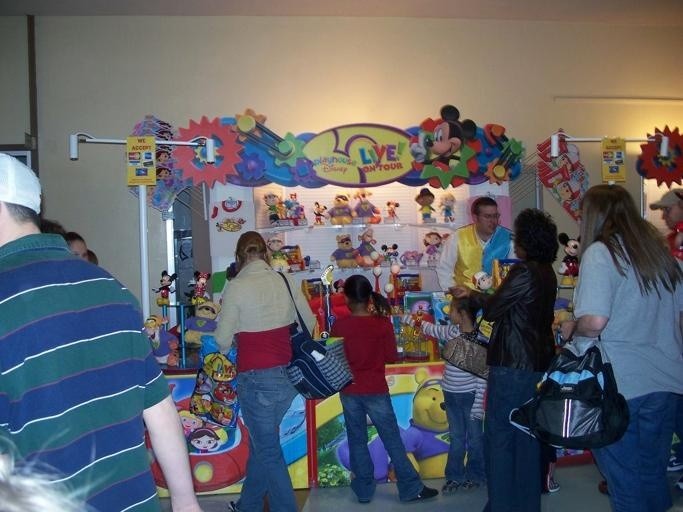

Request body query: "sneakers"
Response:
[460, 478, 477, 489]
[443, 478, 459, 494]
[411, 485, 437, 499]
[667, 449, 682, 472]
[229, 496, 241, 507]
[548, 481, 560, 491]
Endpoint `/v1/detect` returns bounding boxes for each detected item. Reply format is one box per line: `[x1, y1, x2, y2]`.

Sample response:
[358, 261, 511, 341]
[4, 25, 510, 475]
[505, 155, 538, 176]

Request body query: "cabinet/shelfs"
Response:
[129, 102, 527, 499]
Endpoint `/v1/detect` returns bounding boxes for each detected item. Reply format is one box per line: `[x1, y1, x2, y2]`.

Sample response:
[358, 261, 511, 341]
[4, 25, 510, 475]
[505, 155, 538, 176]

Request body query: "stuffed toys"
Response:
[331, 234, 359, 267]
[350, 190, 382, 224]
[179, 302, 224, 344]
[353, 229, 384, 267]
[401, 251, 423, 266]
[325, 192, 357, 225]
[146, 316, 183, 367]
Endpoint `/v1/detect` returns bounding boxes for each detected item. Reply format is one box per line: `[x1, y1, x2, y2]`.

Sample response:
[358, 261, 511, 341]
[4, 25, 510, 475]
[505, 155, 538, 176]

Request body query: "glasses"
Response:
[479, 211, 499, 218]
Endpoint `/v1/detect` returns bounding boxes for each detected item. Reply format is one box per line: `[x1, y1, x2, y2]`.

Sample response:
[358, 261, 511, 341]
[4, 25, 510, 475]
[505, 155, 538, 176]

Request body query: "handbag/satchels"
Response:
[512, 323, 632, 453]
[441, 312, 495, 383]
[276, 268, 358, 402]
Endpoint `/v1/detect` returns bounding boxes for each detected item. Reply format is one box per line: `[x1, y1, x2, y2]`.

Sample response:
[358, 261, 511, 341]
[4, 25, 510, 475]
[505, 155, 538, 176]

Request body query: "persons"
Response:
[439, 192, 457, 224]
[649, 189, 683, 269]
[188, 428, 221, 455]
[382, 245, 399, 266]
[436, 198, 520, 291]
[330, 275, 440, 501]
[384, 201, 400, 222]
[214, 231, 317, 512]
[445, 209, 558, 512]
[65, 232, 89, 263]
[415, 188, 437, 223]
[262, 194, 308, 225]
[424, 232, 449, 260]
[312, 203, 331, 226]
[557, 186, 682, 512]
[267, 237, 321, 272]
[172, 395, 206, 436]
[397, 297, 490, 492]
[2, 152, 208, 512]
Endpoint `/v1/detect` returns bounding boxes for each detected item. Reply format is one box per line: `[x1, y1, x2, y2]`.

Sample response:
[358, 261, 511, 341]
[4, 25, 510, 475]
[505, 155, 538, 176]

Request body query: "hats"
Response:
[1, 153, 43, 216]
[649, 188, 683, 210]
